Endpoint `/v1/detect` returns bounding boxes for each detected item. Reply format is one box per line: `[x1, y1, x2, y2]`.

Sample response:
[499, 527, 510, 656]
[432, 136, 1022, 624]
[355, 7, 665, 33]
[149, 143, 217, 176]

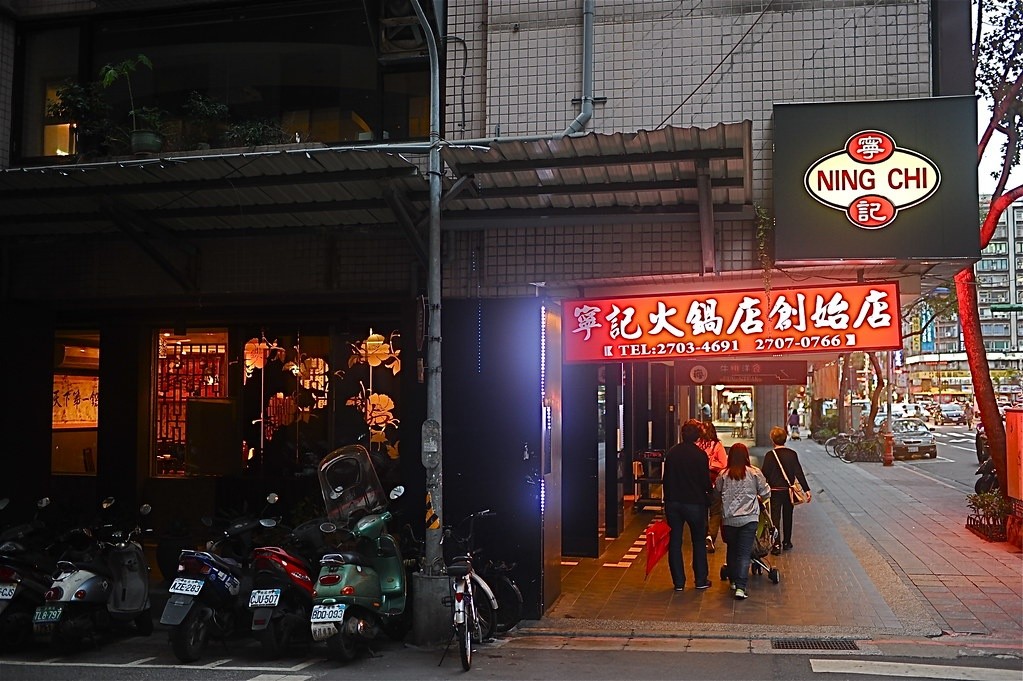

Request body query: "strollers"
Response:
[720, 494, 779, 584]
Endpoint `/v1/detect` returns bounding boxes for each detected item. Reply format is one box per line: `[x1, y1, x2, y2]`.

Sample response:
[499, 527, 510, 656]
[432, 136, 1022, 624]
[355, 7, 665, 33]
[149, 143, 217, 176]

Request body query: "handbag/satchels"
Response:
[708, 440, 724, 472]
[789, 484, 804, 505]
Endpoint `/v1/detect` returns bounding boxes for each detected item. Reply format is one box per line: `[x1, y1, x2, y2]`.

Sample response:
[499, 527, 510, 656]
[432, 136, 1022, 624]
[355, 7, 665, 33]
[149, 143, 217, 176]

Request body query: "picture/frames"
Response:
[51, 369, 101, 431]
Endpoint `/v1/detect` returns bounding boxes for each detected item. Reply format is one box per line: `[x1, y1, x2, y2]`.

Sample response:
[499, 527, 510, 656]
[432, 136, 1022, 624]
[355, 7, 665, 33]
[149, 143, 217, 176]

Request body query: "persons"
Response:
[915, 406, 921, 418]
[762, 426, 811, 554]
[902, 405, 908, 417]
[663, 420, 713, 589]
[720, 400, 748, 422]
[716, 443, 771, 597]
[963, 402, 974, 431]
[701, 403, 710, 419]
[697, 422, 727, 552]
[790, 409, 800, 429]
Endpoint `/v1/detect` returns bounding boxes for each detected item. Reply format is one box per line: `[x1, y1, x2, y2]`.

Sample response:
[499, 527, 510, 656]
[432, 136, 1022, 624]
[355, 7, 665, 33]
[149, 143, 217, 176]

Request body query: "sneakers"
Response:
[735, 588, 747, 598]
[729, 582, 736, 589]
[706, 535, 715, 553]
[696, 580, 710, 589]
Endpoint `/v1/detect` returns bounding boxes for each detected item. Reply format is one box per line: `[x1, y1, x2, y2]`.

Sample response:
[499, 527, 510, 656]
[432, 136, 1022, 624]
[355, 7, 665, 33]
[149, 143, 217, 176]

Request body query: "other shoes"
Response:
[771, 544, 780, 554]
[782, 543, 793, 548]
[674, 585, 682, 590]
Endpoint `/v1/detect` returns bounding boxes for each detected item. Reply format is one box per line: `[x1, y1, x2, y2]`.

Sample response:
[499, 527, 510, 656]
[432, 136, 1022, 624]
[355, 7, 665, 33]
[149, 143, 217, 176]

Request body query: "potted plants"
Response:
[42, 53, 174, 154]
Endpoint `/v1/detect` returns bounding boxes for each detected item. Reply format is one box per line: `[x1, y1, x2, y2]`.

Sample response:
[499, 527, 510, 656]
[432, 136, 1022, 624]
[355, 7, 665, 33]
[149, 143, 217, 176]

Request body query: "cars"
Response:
[933, 404, 968, 426]
[822, 399, 938, 427]
[997, 402, 1014, 418]
[878, 417, 937, 459]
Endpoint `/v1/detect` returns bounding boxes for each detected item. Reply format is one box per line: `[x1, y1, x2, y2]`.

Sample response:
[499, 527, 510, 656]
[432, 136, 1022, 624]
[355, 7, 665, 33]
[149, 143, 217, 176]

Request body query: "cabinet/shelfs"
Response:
[632, 448, 669, 514]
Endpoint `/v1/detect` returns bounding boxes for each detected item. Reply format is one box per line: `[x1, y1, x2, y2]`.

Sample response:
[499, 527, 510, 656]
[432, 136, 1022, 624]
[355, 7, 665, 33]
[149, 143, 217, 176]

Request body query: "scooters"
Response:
[1, 483, 342, 668]
[309, 444, 410, 663]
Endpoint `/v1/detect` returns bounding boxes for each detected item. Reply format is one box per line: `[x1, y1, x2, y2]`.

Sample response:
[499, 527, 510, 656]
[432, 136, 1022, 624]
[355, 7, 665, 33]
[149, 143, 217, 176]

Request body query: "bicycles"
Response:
[825, 428, 885, 465]
[404, 523, 524, 634]
[439, 508, 501, 671]
